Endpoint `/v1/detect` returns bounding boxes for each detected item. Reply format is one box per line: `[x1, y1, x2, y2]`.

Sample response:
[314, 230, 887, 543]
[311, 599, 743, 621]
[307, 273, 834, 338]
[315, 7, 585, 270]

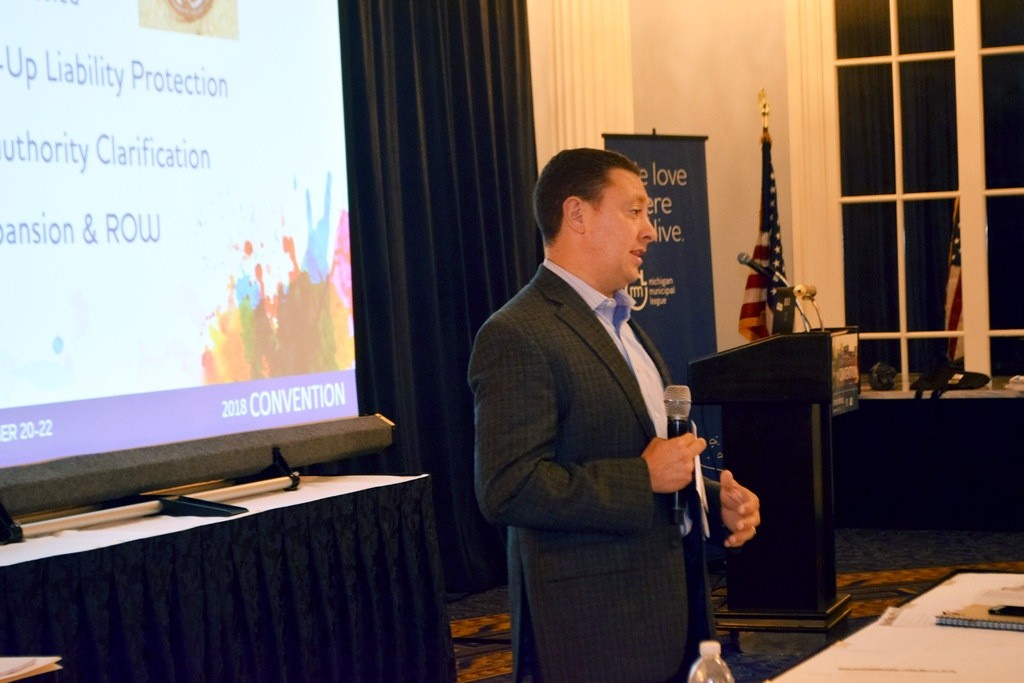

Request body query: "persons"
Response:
[468, 148, 760, 683]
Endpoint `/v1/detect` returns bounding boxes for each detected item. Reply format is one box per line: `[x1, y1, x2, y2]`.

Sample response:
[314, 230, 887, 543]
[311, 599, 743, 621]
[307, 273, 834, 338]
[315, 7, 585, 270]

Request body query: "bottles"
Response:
[690, 641, 734, 683]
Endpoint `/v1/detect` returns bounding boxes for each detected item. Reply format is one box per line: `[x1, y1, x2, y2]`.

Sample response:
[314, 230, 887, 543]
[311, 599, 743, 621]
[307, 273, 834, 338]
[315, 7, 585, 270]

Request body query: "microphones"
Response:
[736, 252, 780, 284]
[662, 384, 692, 526]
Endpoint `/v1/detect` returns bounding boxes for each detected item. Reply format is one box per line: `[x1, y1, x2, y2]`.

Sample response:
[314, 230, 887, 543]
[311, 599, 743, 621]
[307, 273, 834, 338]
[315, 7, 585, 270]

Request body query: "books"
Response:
[935, 604, 1024, 632]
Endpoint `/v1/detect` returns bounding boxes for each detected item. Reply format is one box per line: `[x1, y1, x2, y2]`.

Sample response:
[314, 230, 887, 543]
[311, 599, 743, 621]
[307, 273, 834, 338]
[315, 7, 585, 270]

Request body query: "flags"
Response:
[943, 191, 964, 362]
[738, 103, 787, 344]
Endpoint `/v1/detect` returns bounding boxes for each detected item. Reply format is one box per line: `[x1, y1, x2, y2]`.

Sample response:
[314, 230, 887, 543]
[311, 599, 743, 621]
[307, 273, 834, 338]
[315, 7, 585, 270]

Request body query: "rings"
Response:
[749, 526, 757, 534]
